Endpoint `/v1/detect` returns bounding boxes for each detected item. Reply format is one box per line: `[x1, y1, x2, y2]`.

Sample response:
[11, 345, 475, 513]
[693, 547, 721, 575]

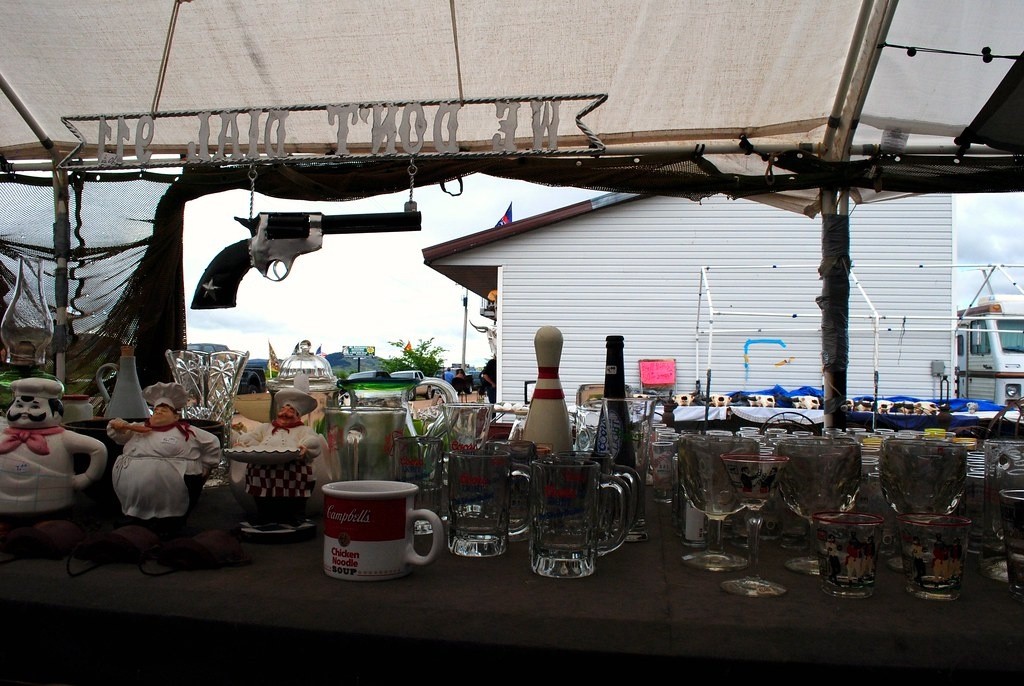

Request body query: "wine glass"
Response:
[677, 431, 967, 600]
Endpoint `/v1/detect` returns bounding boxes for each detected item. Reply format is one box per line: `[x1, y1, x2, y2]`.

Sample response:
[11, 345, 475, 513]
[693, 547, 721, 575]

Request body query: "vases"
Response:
[163, 349, 251, 489]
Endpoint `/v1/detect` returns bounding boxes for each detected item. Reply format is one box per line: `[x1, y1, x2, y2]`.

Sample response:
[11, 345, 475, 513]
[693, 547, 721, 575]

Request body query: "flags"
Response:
[267, 344, 280, 376]
[495, 202, 512, 227]
[404, 342, 411, 352]
[294, 343, 299, 355]
[316, 346, 321, 354]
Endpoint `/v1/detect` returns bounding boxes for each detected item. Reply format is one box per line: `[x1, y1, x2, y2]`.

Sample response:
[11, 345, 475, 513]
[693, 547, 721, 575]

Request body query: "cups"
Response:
[324, 402, 1024, 600]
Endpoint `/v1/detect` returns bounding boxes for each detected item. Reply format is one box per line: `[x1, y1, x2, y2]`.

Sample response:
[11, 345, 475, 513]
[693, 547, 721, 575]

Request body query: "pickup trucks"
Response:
[188, 342, 267, 395]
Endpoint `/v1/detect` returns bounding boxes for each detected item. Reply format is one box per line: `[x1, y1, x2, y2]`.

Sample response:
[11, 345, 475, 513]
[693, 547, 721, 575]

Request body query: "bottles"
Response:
[163, 347, 248, 491]
[60, 396, 94, 423]
[0, 251, 65, 418]
[595, 336, 634, 467]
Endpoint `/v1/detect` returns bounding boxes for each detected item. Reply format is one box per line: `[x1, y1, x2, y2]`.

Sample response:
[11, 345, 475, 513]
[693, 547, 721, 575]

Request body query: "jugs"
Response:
[95, 346, 151, 417]
[329, 370, 462, 478]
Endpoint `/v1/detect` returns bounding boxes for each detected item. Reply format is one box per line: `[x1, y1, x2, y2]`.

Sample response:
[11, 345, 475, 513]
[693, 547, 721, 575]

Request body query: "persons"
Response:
[233, 387, 322, 530]
[483, 353, 496, 420]
[0, 378, 108, 532]
[479, 367, 487, 395]
[342, 346, 352, 354]
[107, 382, 222, 542]
[456, 370, 467, 403]
[444, 367, 454, 384]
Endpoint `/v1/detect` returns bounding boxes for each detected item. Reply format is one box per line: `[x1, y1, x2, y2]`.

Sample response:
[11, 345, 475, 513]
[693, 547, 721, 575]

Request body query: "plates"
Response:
[222, 444, 300, 465]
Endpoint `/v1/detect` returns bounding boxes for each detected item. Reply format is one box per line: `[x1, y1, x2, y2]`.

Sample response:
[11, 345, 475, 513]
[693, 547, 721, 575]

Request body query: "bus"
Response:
[955, 294, 1024, 414]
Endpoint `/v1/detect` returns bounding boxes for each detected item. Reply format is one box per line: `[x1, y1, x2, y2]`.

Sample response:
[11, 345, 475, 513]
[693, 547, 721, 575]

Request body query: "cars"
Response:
[433, 366, 474, 394]
[338, 371, 391, 404]
[388, 369, 432, 402]
[467, 371, 483, 390]
